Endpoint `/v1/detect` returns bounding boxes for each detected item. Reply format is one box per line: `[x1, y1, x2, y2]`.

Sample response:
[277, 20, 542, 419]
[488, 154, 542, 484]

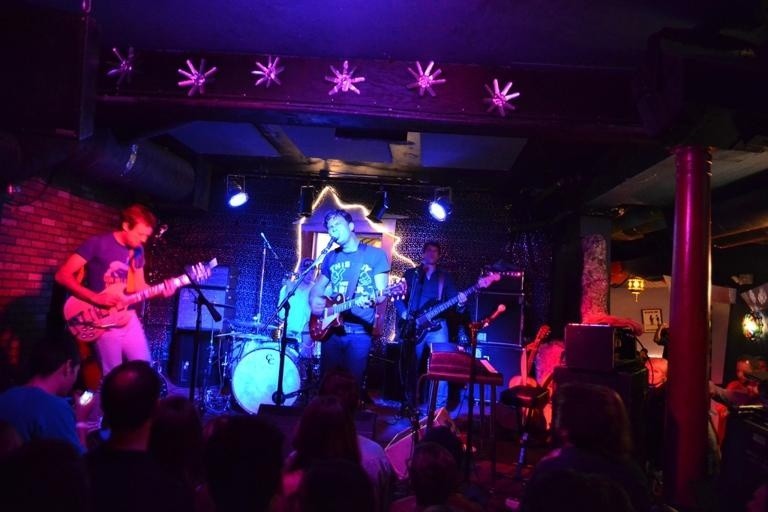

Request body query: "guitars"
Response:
[309, 278, 407, 341]
[61, 258, 218, 343]
[401, 273, 500, 344]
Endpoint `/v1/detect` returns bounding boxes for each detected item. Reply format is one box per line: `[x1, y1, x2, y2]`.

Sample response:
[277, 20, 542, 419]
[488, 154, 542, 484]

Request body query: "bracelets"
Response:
[457, 302, 464, 307]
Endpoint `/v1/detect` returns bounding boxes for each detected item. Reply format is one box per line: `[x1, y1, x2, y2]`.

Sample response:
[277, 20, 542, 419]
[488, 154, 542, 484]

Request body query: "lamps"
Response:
[628, 276, 646, 303]
[743, 313, 767, 343]
[224, 172, 490, 223]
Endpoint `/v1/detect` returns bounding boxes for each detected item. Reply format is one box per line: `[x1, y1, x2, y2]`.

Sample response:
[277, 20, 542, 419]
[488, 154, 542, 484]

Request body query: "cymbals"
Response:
[214, 331, 273, 343]
[232, 319, 278, 330]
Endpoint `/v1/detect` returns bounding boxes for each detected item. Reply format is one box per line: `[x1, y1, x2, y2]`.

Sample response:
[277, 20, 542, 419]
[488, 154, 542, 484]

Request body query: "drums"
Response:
[231, 342, 303, 417]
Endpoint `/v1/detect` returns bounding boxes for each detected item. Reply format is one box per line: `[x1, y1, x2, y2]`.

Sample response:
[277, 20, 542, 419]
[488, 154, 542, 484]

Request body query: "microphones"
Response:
[414, 260, 425, 279]
[322, 231, 339, 255]
[153, 224, 168, 246]
[490, 304, 506, 321]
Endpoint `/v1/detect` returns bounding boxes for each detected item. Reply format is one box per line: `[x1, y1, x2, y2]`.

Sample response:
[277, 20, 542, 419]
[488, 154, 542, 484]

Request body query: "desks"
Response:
[427, 359, 503, 496]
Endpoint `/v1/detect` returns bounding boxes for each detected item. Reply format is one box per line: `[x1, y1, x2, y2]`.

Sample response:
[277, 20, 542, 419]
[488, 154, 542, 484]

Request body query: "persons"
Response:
[1, 320, 768, 512]
[306, 210, 390, 385]
[55, 205, 176, 430]
[393, 241, 467, 411]
[277, 257, 315, 344]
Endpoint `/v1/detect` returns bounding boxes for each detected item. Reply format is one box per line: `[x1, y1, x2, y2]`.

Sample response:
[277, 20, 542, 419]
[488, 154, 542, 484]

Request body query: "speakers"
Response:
[253, 404, 376, 444]
[564, 324, 636, 371]
[555, 367, 648, 464]
[383, 407, 460, 481]
